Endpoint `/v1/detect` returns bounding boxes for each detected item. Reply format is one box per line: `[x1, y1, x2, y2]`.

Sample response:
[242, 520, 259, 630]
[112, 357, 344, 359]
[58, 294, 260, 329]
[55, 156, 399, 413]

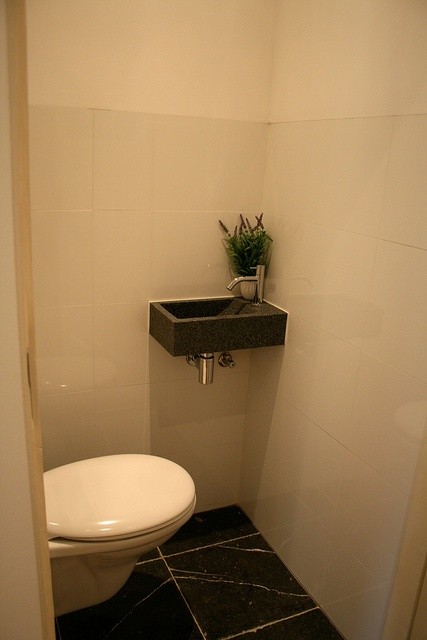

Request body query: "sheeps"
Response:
[227, 265, 265, 302]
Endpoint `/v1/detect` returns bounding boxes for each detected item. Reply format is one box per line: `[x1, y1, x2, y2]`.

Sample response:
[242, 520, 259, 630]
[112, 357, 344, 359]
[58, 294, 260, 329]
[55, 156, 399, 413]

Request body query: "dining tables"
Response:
[216, 210, 269, 303]
